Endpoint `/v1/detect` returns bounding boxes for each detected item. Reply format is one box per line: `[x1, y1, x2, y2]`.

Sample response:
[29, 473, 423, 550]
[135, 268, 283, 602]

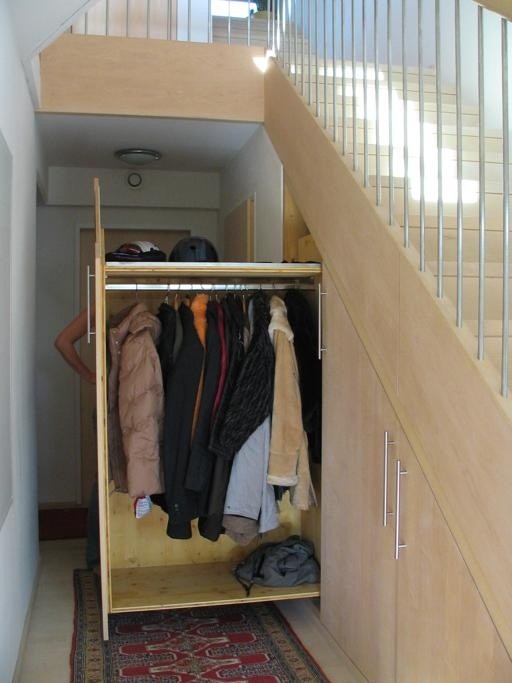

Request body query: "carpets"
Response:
[71, 565, 332, 683]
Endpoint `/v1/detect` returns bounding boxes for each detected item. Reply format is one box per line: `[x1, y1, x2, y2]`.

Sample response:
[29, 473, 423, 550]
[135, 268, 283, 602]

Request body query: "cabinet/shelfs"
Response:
[84, 176, 319, 641]
[321, 266, 512, 683]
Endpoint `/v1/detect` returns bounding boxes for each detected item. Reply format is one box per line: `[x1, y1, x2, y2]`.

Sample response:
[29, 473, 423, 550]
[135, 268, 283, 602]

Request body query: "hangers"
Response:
[128, 280, 306, 312]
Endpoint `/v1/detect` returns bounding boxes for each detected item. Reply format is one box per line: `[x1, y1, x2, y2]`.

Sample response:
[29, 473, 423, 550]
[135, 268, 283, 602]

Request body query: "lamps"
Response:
[114, 149, 162, 167]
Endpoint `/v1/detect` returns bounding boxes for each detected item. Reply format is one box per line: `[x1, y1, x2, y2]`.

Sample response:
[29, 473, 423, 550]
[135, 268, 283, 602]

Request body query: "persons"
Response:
[54, 300, 100, 579]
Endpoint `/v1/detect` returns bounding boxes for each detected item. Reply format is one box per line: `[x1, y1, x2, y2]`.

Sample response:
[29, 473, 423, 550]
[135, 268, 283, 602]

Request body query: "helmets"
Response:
[168, 237, 219, 262]
